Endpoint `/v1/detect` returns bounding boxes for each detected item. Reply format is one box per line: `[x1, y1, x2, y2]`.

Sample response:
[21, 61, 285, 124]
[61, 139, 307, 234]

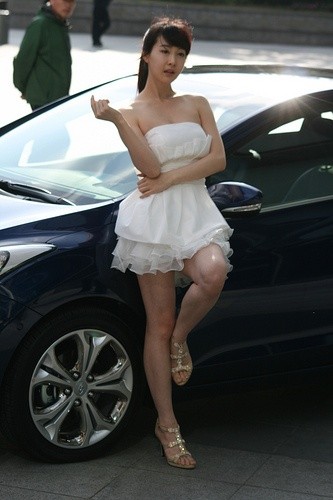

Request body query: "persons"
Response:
[12, 0, 112, 113]
[90, 19, 233, 469]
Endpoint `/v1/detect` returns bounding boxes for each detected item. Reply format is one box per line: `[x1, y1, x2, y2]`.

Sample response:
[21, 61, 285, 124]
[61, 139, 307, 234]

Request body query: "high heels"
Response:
[171, 336, 193, 386]
[154, 419, 195, 469]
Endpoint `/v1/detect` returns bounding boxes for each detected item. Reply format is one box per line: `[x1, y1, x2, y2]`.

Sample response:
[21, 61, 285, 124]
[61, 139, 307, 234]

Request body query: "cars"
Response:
[0, 63, 333, 462]
